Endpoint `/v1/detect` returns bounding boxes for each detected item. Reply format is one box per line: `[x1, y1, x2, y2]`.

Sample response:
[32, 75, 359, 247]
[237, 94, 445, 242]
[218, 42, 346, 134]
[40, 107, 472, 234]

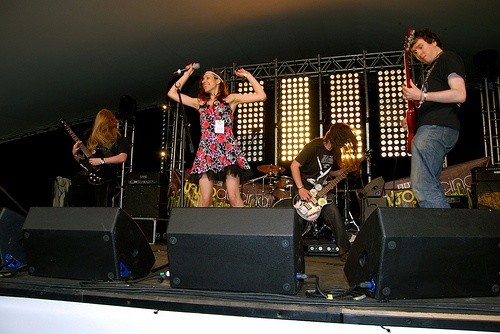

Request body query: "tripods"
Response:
[305, 178, 361, 237]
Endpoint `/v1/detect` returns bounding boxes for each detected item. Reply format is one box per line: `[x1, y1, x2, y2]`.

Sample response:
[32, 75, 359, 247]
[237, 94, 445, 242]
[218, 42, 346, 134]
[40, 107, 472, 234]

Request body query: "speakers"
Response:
[344, 207, 500, 304]
[125, 184, 161, 237]
[0, 208, 27, 272]
[166, 208, 305, 295]
[23, 206, 154, 284]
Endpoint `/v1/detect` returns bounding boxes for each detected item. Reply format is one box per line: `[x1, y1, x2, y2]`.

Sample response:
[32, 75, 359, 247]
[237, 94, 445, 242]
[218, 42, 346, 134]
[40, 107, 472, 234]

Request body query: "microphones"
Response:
[174, 63, 201, 74]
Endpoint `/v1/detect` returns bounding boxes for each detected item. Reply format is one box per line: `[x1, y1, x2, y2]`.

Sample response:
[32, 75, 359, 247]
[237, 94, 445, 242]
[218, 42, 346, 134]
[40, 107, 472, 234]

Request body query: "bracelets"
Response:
[423, 92, 427, 101]
[298, 185, 304, 189]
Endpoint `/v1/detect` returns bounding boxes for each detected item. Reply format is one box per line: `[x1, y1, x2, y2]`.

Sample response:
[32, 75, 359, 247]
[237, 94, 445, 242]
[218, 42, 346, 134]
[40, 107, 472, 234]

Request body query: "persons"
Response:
[290, 122, 358, 256]
[167, 63, 268, 207]
[402, 29, 467, 209]
[72, 109, 128, 208]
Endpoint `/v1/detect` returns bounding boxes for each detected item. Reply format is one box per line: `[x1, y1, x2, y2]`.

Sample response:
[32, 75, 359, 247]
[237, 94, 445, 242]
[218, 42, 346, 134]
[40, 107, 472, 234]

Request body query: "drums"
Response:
[270, 197, 294, 208]
[272, 176, 294, 199]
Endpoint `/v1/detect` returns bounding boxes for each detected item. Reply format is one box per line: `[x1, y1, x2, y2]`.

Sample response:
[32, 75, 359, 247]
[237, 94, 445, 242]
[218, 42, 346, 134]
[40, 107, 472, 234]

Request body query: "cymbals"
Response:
[257, 165, 285, 173]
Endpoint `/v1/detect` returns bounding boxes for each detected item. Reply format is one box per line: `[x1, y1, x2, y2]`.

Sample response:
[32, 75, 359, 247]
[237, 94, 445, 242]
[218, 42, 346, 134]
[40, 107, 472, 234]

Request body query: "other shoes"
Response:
[339, 243, 353, 261]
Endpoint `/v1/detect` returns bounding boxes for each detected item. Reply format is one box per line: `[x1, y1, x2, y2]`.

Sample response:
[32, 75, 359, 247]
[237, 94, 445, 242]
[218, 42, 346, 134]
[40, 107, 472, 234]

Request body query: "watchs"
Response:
[100, 158, 104, 164]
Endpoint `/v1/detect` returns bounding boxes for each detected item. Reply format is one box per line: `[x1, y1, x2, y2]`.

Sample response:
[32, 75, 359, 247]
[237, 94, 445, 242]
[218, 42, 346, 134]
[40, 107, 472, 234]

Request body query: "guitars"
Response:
[57, 118, 114, 185]
[293, 148, 374, 221]
[403, 28, 420, 154]
